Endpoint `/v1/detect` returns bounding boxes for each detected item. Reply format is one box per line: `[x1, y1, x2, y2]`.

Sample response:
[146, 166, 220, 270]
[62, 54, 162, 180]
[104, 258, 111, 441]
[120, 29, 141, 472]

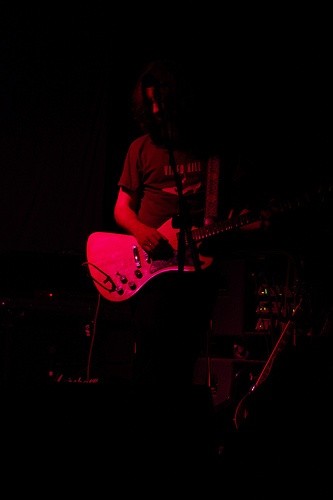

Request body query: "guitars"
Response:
[84, 175, 332, 303]
[233, 304, 303, 432]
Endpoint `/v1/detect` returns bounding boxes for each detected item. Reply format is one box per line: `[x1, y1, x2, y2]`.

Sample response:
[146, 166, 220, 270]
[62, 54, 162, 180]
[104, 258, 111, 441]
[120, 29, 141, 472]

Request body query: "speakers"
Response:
[194, 358, 270, 406]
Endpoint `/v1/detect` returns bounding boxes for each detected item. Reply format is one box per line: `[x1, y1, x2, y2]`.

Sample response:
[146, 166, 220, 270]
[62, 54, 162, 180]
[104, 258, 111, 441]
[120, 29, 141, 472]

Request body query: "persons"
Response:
[111, 60, 230, 390]
